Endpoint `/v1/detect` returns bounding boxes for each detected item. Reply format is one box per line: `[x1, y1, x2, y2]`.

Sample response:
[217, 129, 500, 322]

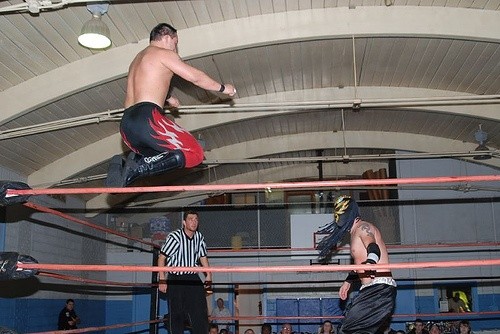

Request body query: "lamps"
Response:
[473, 123, 493, 160]
[192, 133, 209, 168]
[78, 0, 113, 50]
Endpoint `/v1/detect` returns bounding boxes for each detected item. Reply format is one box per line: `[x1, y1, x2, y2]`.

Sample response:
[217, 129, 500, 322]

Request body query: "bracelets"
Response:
[219, 84, 225, 92]
[166, 94, 172, 100]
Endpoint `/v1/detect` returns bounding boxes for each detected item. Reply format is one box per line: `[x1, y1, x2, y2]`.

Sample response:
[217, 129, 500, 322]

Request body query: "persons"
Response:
[158, 211, 213, 334]
[57, 298, 81, 330]
[105, 22, 237, 191]
[244, 329, 255, 334]
[320, 320, 334, 334]
[458, 319, 471, 334]
[384, 318, 440, 334]
[448, 293, 470, 313]
[279, 323, 293, 334]
[315, 195, 396, 334]
[208, 298, 234, 334]
[261, 323, 272, 334]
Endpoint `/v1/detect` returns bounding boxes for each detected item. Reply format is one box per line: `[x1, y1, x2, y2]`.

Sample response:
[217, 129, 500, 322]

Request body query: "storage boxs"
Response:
[111, 215, 173, 246]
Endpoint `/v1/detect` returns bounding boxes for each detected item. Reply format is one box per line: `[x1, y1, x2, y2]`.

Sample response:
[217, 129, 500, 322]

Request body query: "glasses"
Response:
[283, 327, 290, 330]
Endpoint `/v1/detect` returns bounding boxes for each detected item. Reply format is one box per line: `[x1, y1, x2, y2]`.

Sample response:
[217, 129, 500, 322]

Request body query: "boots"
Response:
[106, 148, 186, 196]
